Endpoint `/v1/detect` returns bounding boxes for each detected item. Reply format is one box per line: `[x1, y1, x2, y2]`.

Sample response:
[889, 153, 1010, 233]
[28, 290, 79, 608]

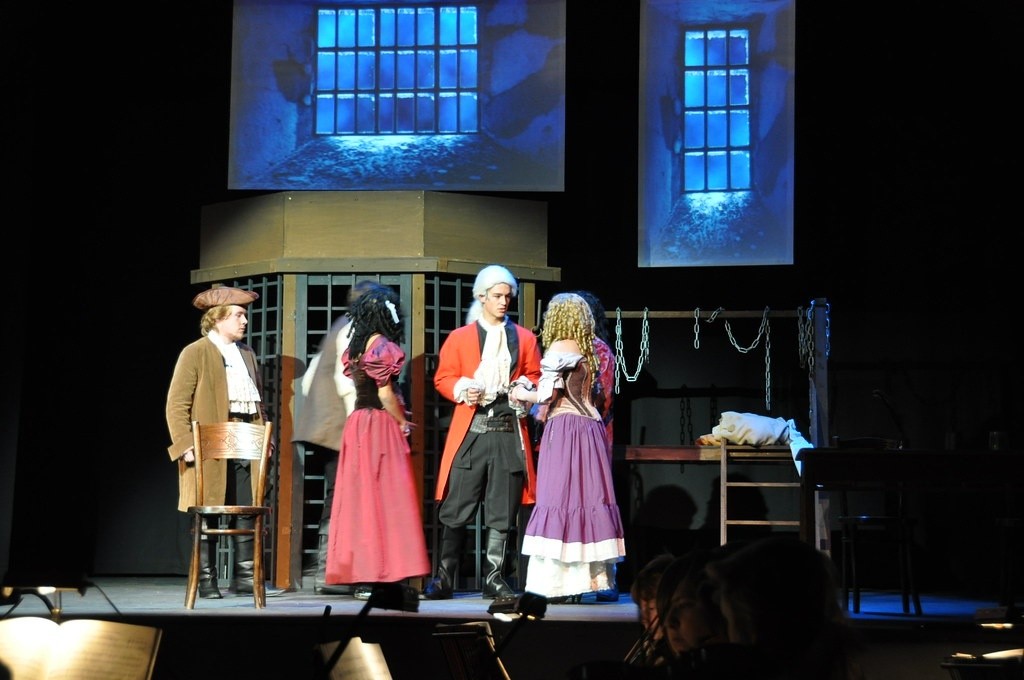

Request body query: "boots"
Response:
[229, 517, 287, 597]
[482, 527, 514, 600]
[192, 516, 223, 599]
[416, 525, 467, 599]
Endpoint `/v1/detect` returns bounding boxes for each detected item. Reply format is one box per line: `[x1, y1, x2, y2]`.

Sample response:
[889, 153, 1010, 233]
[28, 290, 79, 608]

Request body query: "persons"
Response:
[166, 285, 287, 600]
[291, 280, 429, 600]
[630, 537, 858, 680]
[422, 265, 542, 601]
[512, 290, 626, 602]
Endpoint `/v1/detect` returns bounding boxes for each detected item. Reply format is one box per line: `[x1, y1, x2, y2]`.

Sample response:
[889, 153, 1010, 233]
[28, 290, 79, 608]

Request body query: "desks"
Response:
[800, 448, 1024, 494]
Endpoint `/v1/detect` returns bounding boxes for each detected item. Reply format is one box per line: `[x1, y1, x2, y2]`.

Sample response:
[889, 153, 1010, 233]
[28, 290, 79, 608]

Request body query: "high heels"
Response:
[546, 593, 583, 606]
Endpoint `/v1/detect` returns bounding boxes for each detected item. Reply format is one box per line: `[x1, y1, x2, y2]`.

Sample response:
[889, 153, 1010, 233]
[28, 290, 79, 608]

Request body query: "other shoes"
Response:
[354, 583, 378, 601]
[317, 575, 351, 595]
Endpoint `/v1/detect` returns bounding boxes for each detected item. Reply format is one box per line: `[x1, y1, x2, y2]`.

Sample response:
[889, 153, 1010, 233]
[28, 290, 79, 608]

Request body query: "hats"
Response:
[193, 286, 259, 309]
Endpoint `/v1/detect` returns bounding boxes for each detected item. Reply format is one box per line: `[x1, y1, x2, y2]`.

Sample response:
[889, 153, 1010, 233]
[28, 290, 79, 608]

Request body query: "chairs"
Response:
[994, 479, 1024, 610]
[833, 436, 922, 615]
[184, 420, 273, 609]
